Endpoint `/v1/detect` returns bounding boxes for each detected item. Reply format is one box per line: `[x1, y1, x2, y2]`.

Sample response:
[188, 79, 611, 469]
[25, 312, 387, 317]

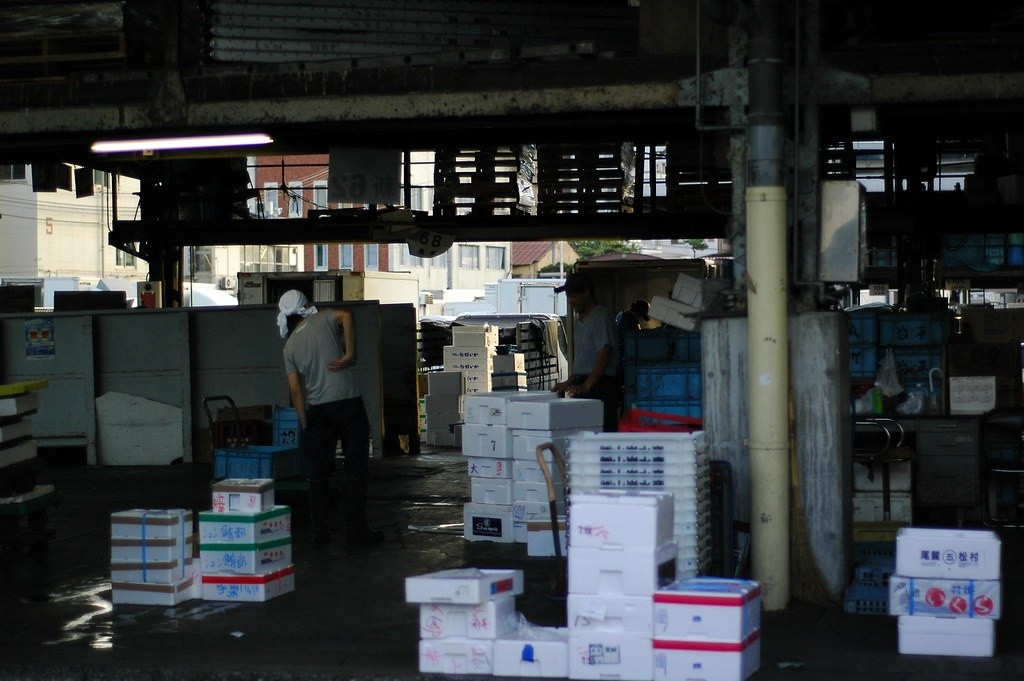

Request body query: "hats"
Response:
[635, 299, 650, 321]
[555, 273, 594, 293]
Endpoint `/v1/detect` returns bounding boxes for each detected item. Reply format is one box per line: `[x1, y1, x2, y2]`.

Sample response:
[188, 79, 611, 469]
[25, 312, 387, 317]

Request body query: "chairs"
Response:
[980, 406, 1024, 529]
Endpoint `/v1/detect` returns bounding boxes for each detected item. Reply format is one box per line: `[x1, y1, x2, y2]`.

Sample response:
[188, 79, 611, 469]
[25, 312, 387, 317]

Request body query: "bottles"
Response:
[873, 382, 882, 413]
[915, 384, 926, 416]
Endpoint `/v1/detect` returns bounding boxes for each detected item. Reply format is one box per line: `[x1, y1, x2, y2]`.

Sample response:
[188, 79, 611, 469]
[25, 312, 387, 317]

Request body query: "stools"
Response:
[855, 416, 910, 523]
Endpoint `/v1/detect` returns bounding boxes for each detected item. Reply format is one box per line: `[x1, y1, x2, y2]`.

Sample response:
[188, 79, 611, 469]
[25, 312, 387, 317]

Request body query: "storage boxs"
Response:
[843, 175, 1023, 659]
[0, 378, 66, 517]
[110, 404, 301, 607]
[649, 276, 722, 334]
[403, 324, 762, 681]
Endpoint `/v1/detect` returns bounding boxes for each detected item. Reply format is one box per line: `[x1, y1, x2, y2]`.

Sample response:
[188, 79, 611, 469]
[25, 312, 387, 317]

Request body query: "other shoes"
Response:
[348, 530, 383, 545]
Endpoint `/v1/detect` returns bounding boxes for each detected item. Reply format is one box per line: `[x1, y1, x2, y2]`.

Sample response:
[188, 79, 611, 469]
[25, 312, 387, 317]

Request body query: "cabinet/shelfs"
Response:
[852, 409, 992, 529]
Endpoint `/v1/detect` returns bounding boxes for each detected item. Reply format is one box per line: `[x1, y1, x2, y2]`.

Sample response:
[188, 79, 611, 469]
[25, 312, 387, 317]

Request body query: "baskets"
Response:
[842, 565, 892, 616]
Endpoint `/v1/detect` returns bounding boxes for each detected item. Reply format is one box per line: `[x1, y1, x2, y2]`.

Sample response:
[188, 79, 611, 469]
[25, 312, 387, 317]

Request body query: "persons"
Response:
[277, 289, 383, 548]
[616, 299, 650, 340]
[553, 272, 621, 432]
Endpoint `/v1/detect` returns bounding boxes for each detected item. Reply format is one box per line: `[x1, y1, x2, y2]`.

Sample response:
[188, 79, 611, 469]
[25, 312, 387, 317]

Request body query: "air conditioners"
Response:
[221, 277, 236, 290]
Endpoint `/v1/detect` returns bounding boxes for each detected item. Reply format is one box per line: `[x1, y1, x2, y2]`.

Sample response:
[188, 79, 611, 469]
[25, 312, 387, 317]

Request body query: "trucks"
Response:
[232, 267, 419, 326]
